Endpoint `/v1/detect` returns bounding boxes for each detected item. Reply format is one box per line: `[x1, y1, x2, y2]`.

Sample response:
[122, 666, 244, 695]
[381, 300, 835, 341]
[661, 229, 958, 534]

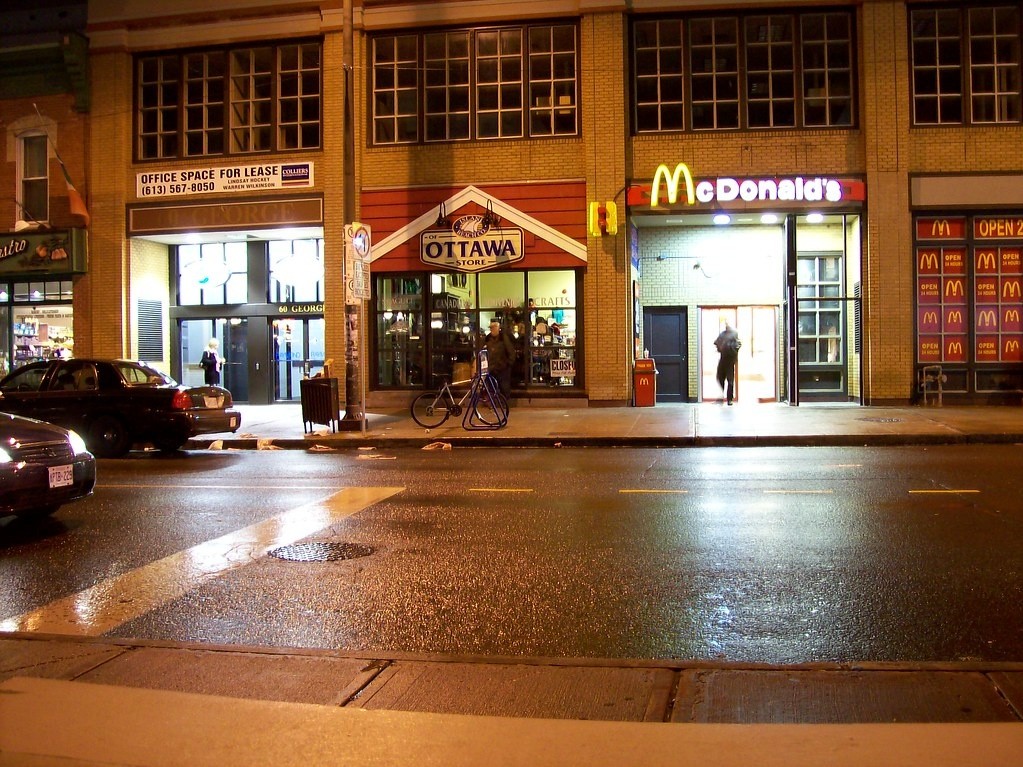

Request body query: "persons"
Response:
[714, 322, 741, 405]
[60, 347, 72, 357]
[482, 322, 515, 407]
[199, 338, 220, 387]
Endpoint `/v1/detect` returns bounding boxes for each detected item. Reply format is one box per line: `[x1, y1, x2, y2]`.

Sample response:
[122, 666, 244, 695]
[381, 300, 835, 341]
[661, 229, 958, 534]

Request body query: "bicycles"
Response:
[411, 371, 508, 428]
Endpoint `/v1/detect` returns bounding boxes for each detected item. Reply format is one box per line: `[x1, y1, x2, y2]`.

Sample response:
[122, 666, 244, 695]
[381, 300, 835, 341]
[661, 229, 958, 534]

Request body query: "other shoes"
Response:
[712, 400, 724, 404]
[727, 400, 733, 405]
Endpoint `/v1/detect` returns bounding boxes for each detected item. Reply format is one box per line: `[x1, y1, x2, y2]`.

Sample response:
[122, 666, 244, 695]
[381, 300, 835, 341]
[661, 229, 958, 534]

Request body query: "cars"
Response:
[0, 359, 241, 455]
[0, 412, 97, 518]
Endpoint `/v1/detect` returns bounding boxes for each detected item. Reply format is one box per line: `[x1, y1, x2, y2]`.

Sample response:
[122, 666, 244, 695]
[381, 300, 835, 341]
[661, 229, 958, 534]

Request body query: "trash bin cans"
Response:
[634, 357, 660, 408]
[300, 378, 341, 434]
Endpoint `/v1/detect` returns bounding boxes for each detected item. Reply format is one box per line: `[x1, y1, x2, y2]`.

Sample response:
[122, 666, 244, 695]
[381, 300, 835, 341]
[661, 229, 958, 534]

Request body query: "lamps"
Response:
[435, 201, 451, 228]
[482, 199, 497, 229]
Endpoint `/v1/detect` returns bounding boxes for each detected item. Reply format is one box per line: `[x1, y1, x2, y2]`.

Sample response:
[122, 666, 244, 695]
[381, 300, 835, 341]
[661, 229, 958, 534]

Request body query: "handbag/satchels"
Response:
[198, 350, 210, 370]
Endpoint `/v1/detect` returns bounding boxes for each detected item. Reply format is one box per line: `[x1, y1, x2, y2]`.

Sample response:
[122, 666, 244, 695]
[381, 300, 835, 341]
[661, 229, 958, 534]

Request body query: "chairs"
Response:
[85, 376, 96, 389]
[59, 375, 77, 389]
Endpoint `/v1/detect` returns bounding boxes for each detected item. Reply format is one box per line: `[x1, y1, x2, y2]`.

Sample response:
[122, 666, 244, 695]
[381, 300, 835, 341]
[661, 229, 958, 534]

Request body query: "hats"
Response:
[488, 322, 500, 328]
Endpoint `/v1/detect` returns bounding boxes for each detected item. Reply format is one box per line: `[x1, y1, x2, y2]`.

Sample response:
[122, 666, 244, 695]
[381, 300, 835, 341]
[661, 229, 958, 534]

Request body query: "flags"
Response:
[57, 155, 89, 226]
